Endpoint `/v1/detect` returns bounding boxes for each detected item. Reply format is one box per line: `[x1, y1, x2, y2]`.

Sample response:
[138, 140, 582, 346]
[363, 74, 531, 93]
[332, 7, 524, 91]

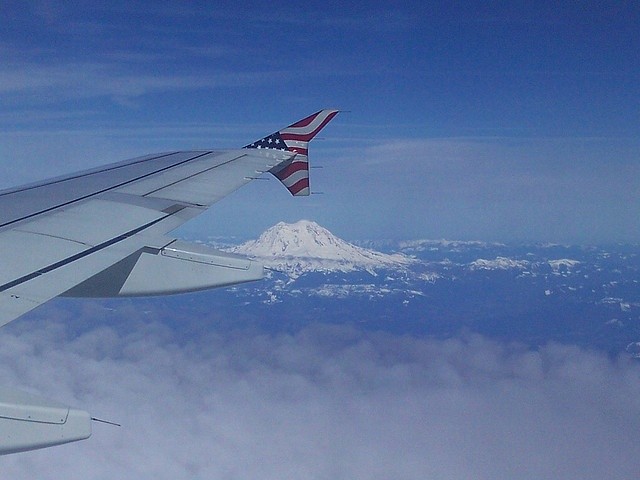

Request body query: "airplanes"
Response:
[0, 109, 339, 455]
